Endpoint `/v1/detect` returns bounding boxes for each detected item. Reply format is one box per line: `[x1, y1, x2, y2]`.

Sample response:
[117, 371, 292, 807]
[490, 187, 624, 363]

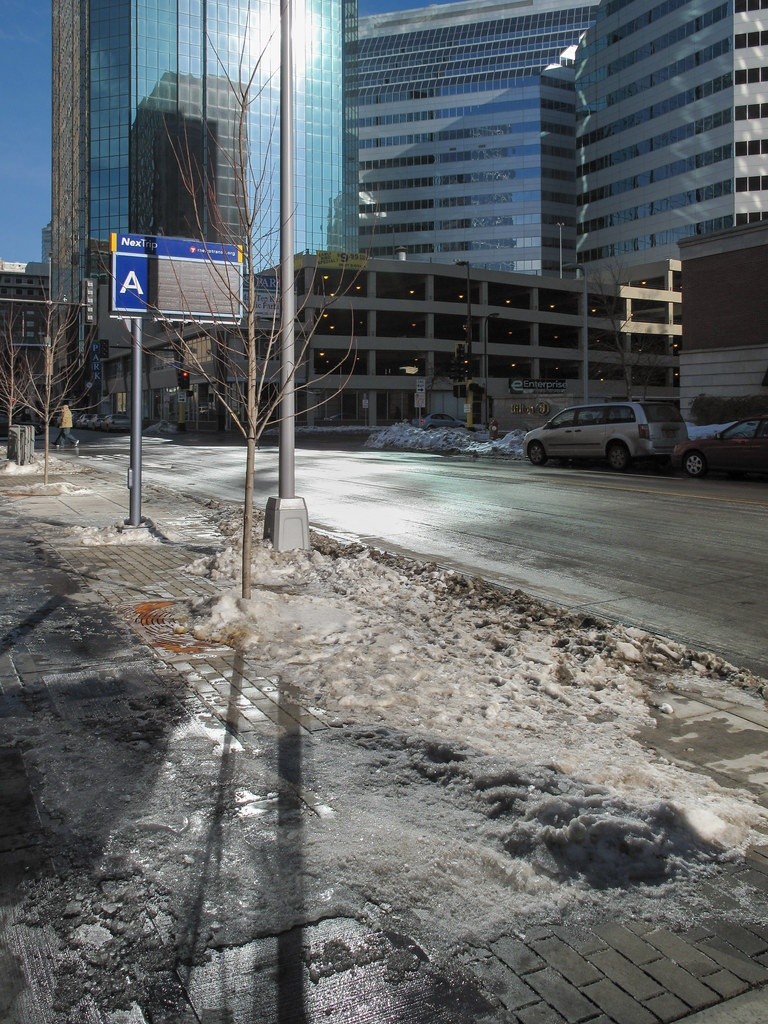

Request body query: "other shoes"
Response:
[51, 442, 59, 447]
[73, 440, 79, 447]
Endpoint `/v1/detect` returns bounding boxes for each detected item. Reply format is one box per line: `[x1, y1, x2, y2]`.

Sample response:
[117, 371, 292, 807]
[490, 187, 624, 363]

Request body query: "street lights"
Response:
[556, 221, 565, 279]
[455, 261, 474, 431]
[484, 312, 500, 429]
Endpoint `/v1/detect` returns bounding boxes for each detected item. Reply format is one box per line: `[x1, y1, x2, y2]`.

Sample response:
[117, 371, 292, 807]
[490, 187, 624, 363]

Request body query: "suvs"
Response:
[523, 400, 690, 472]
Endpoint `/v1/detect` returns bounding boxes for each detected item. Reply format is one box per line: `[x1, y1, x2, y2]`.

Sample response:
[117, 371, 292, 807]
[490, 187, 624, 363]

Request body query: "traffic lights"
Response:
[179, 370, 190, 390]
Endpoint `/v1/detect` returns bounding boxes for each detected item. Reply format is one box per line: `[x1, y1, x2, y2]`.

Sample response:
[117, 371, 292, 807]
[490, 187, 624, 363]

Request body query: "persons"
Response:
[51, 405, 80, 447]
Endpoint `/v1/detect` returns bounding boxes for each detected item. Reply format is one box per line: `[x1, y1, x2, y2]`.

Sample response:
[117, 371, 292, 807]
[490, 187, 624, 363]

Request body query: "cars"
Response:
[412, 413, 466, 431]
[0, 414, 45, 438]
[87, 413, 106, 432]
[670, 416, 768, 480]
[100, 414, 132, 433]
[57, 411, 78, 429]
[75, 414, 93, 430]
